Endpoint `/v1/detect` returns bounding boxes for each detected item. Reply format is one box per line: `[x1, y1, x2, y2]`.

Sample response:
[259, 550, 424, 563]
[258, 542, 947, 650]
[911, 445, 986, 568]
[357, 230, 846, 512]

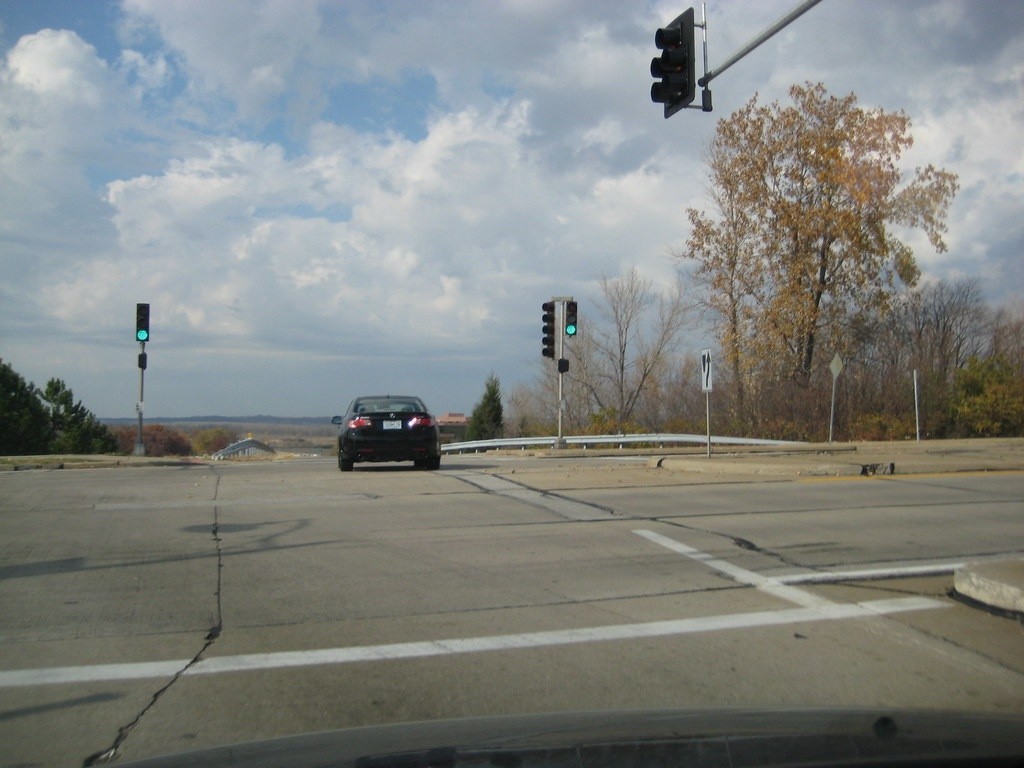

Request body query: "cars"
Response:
[332, 395, 441, 472]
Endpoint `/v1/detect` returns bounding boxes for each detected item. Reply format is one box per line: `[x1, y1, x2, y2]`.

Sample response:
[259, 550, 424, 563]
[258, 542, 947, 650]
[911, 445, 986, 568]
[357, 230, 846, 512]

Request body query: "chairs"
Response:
[401, 406, 414, 413]
[359, 406, 376, 413]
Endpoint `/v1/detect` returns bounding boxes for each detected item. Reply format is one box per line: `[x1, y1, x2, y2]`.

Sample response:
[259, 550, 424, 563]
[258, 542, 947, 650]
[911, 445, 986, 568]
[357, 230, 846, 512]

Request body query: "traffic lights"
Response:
[542, 301, 555, 359]
[137, 303, 150, 341]
[650, 7, 695, 119]
[566, 302, 578, 335]
